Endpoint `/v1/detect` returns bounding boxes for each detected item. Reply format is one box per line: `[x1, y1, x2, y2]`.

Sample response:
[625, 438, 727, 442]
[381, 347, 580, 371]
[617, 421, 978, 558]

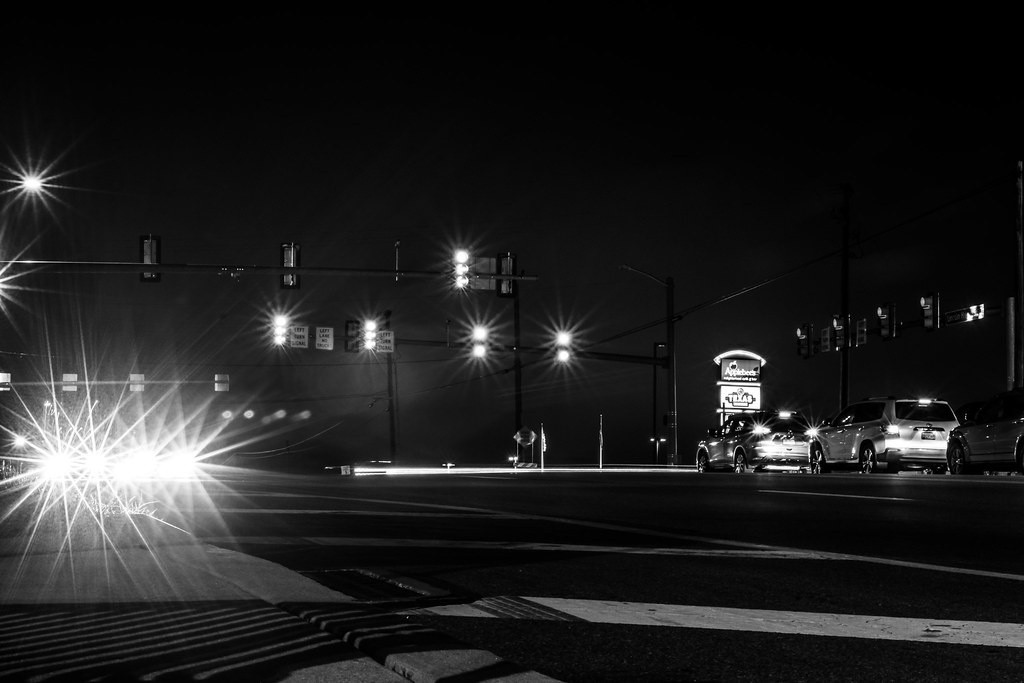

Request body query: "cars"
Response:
[695, 408, 820, 475]
[808, 395, 960, 476]
[946, 386, 1024, 475]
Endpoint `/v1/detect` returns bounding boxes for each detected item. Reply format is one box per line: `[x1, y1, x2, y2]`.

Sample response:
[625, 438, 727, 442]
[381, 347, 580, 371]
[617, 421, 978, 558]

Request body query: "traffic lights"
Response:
[798, 323, 810, 359]
[879, 312, 891, 339]
[923, 294, 934, 330]
[449, 247, 473, 293]
[362, 319, 378, 351]
[473, 323, 490, 360]
[553, 328, 573, 362]
[270, 313, 289, 348]
